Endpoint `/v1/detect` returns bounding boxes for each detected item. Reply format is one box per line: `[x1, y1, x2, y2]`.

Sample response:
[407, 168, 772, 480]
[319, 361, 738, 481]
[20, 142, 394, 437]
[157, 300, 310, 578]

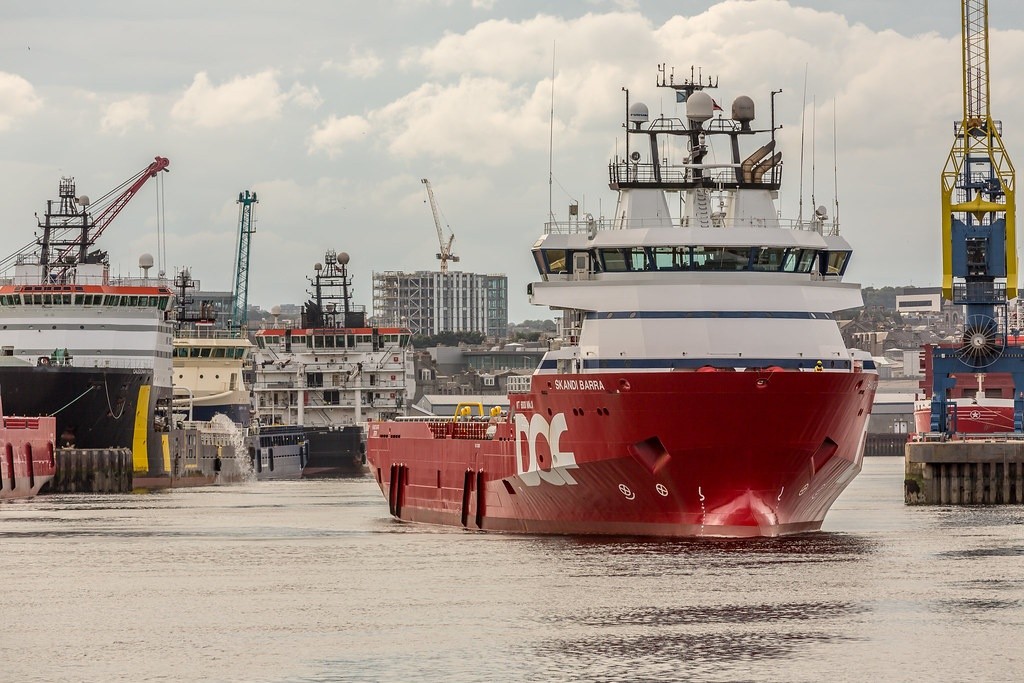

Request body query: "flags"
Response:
[712, 99, 723, 111]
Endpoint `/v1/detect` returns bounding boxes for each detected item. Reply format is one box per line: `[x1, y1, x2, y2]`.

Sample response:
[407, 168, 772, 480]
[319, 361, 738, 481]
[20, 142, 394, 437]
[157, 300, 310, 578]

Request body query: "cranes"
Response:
[420, 179, 458, 271]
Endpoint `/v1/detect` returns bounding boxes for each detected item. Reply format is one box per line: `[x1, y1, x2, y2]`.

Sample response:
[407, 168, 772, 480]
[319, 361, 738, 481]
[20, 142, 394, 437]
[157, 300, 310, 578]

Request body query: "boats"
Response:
[366, 40, 878, 540]
[165, 189, 309, 479]
[248, 248, 416, 472]
[1, 155, 181, 476]
